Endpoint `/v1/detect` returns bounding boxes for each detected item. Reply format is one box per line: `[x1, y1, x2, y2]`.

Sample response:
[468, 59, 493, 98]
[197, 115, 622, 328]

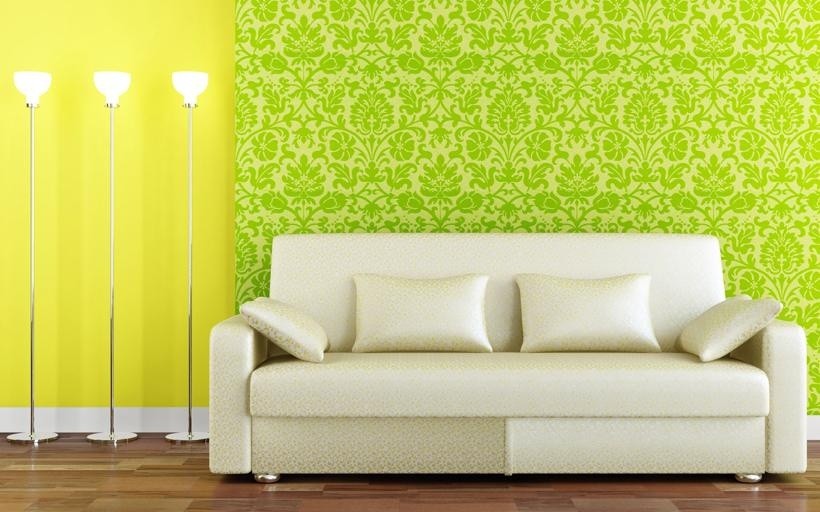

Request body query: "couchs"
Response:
[209, 232, 810, 484]
